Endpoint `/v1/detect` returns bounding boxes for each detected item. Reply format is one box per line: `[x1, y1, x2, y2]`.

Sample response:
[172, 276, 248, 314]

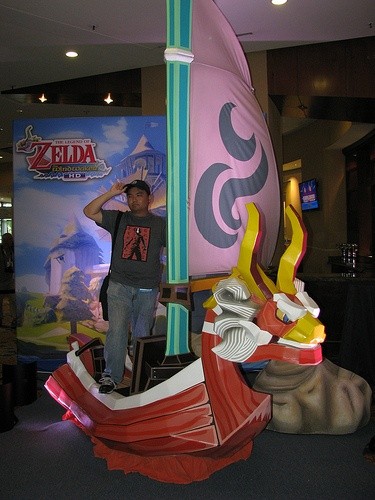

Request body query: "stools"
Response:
[2, 354, 39, 408]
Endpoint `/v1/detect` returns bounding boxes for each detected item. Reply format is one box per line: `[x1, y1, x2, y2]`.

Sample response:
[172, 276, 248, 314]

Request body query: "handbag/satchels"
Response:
[99, 275, 110, 321]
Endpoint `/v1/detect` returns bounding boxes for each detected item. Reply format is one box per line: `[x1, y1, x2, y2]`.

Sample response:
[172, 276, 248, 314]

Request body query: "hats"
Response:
[122, 179, 151, 196]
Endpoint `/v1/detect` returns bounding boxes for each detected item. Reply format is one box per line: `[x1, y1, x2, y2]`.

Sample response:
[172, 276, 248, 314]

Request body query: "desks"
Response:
[293, 271, 375, 383]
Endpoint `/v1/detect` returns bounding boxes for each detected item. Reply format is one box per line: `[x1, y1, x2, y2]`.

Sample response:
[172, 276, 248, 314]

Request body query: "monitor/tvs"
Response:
[299, 178, 320, 212]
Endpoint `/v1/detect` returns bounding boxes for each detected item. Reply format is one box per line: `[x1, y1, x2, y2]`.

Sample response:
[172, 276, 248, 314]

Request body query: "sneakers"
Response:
[99, 374, 118, 394]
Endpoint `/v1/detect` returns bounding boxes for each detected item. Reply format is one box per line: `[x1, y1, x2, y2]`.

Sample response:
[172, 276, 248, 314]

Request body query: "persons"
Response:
[0, 233, 17, 329]
[82, 178, 169, 394]
